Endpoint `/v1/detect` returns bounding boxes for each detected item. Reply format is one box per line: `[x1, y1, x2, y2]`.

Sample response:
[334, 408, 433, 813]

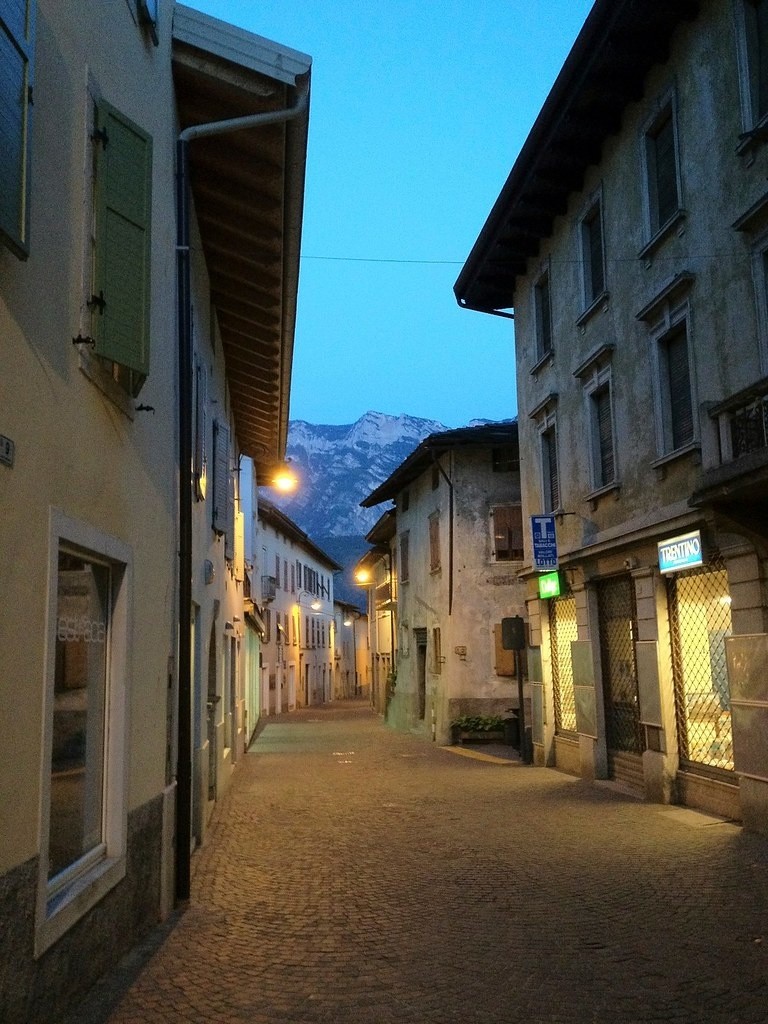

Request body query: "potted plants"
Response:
[449, 715, 504, 739]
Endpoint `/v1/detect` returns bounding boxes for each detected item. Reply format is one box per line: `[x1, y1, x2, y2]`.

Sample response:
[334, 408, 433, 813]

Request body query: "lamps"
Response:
[333, 612, 352, 626]
[298, 590, 321, 610]
